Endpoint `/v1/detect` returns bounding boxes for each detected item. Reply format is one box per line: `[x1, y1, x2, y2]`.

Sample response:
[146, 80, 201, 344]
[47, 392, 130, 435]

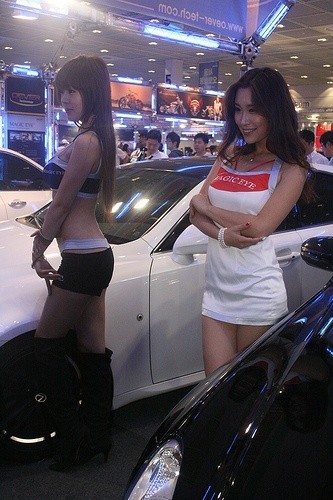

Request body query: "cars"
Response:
[0, 147, 332, 500]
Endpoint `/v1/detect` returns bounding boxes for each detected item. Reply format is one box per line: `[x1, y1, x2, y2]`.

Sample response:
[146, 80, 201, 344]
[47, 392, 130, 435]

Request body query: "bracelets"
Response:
[30, 230, 52, 269]
[218, 227, 229, 249]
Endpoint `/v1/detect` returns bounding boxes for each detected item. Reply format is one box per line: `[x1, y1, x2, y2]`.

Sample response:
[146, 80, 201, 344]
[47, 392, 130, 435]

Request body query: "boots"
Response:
[61, 347, 114, 464]
[10, 336, 65, 462]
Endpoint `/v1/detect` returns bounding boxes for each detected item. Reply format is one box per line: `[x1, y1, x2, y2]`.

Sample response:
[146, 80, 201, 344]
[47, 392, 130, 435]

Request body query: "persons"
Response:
[30, 56, 116, 461]
[55, 129, 332, 166]
[186, 68, 313, 378]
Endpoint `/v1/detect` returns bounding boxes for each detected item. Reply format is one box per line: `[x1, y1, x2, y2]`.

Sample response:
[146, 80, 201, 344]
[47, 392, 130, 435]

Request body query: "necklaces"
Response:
[249, 157, 253, 163]
